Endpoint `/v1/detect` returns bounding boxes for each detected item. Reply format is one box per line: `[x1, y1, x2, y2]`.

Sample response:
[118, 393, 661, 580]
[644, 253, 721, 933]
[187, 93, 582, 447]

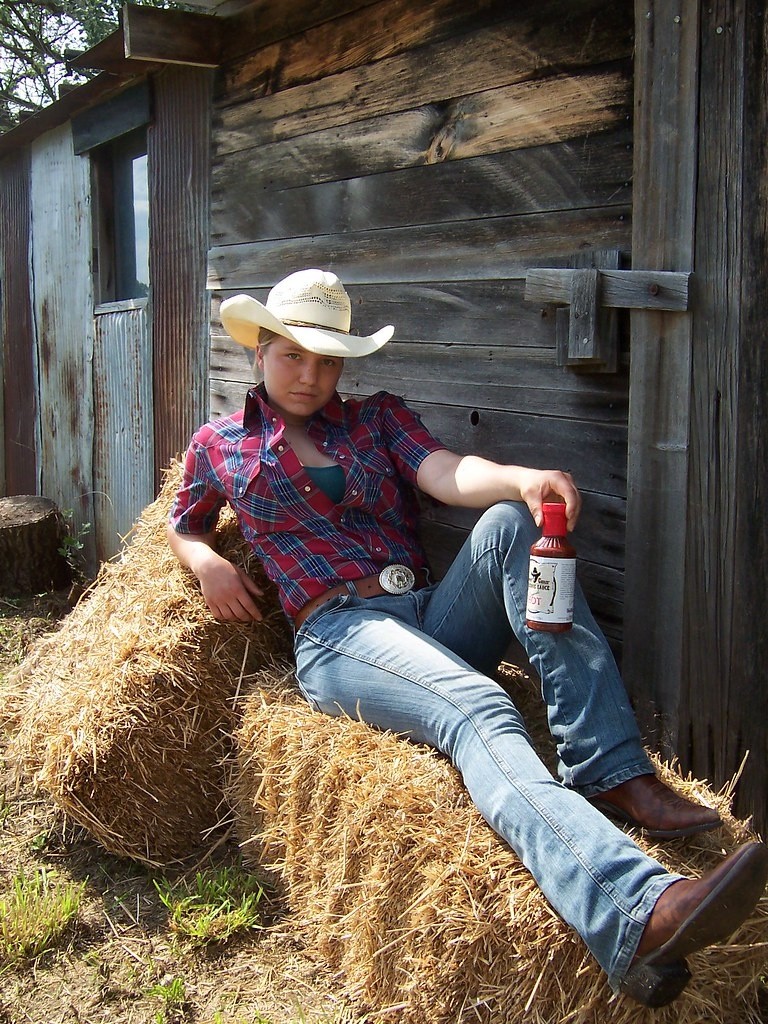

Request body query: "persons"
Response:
[166, 269, 768, 1007]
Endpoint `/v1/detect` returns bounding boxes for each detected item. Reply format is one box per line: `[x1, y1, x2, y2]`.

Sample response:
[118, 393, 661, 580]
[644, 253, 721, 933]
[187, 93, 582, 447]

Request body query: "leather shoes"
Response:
[591, 773, 724, 837]
[618, 840, 768, 1008]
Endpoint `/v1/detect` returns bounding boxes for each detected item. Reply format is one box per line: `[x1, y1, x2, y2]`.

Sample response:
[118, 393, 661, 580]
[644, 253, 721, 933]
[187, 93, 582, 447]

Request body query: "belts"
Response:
[293, 563, 443, 631]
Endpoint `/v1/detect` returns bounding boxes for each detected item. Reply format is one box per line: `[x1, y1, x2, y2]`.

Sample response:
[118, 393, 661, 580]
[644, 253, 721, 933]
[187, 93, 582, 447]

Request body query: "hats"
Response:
[220, 268, 395, 357]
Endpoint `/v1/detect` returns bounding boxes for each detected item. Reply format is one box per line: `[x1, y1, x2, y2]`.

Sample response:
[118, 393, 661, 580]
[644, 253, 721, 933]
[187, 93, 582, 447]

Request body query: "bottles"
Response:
[526, 500, 576, 632]
